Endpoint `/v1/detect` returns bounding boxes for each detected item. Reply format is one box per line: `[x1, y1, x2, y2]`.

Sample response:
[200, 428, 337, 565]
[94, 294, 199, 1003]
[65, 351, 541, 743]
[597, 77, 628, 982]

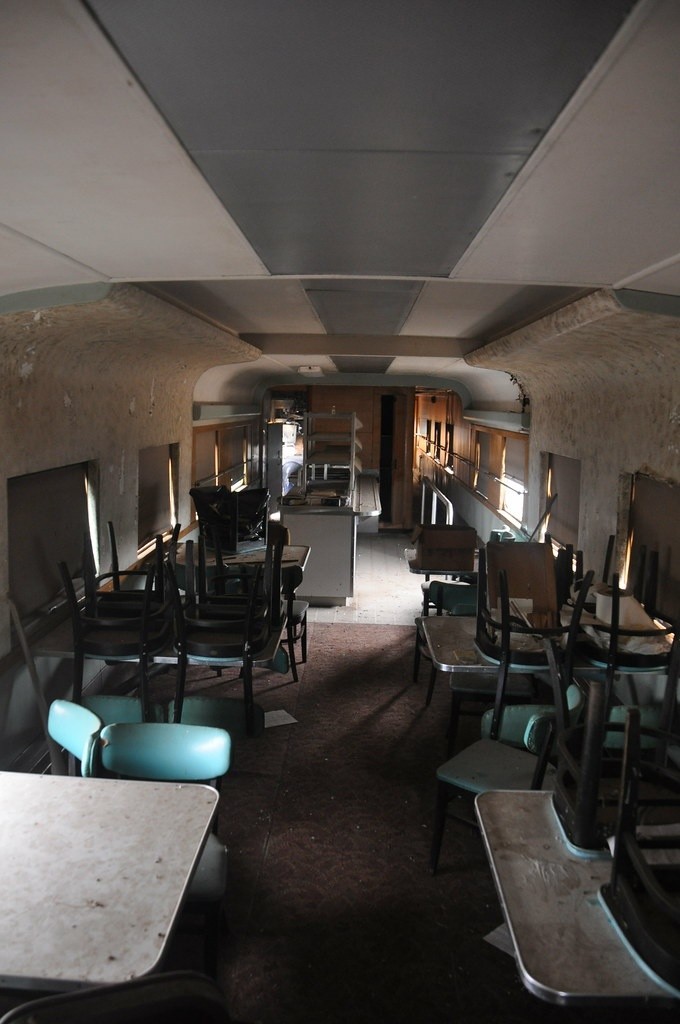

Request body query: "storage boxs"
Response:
[410, 523, 477, 572]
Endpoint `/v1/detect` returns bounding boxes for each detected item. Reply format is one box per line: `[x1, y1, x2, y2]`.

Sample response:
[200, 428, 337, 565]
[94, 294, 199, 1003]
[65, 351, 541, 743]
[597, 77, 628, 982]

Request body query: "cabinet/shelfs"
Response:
[303, 411, 356, 489]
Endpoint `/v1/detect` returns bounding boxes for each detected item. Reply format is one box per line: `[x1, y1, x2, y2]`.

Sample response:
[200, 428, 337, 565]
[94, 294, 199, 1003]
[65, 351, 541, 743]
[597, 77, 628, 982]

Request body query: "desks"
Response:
[143, 544, 310, 572]
[404, 548, 485, 575]
[0, 771, 219, 992]
[474, 790, 680, 1009]
[420, 614, 613, 674]
[29, 604, 288, 668]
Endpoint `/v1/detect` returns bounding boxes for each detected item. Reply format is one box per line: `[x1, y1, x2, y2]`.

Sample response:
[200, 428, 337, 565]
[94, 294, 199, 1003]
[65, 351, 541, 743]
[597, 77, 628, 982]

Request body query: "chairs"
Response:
[0, 485, 680, 1024]
